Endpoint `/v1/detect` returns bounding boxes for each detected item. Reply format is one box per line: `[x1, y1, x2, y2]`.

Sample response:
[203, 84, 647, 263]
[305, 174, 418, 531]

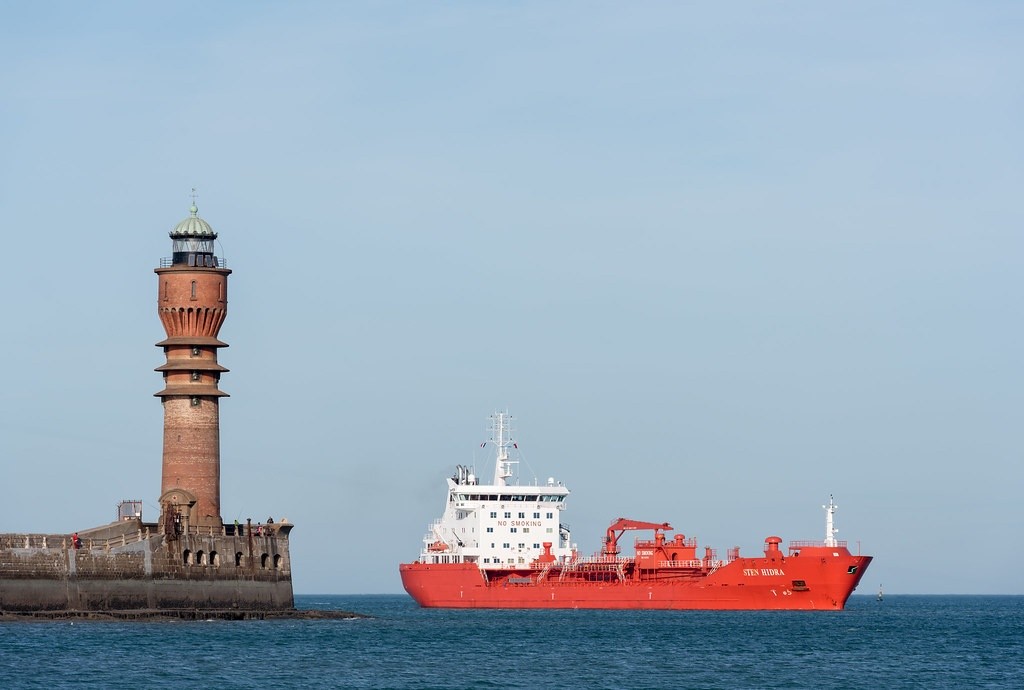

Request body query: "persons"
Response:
[74, 532, 82, 549]
[254, 516, 274, 537]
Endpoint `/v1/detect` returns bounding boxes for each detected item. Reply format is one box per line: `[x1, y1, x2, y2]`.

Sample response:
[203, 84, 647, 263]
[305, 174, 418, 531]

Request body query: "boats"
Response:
[398, 404, 873, 610]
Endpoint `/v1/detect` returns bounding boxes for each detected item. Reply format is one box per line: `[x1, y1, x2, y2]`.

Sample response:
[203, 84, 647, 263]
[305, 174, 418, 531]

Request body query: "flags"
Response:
[480, 443, 486, 448]
